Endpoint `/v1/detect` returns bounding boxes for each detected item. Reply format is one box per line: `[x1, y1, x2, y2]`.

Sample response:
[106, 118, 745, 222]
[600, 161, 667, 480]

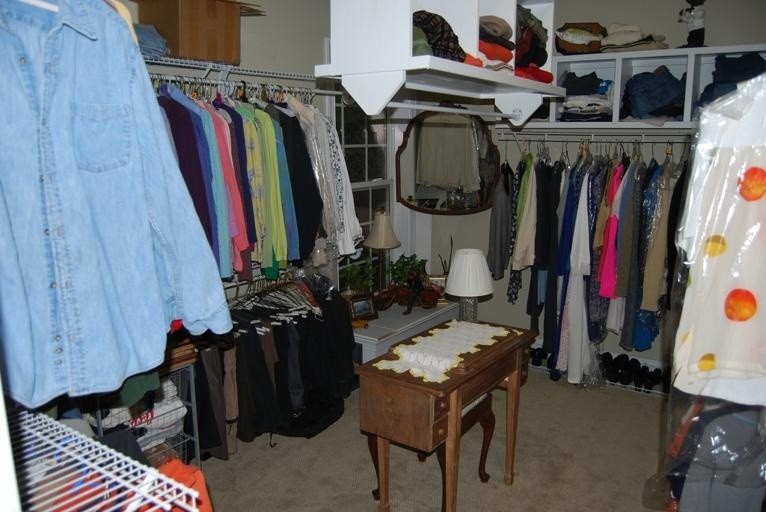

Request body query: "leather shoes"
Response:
[533, 348, 546, 367]
[600, 351, 662, 393]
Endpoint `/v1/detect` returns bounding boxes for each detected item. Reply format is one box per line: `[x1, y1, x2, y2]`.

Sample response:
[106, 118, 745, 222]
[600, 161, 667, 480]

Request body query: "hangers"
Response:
[220, 267, 334, 336]
[149, 73, 316, 111]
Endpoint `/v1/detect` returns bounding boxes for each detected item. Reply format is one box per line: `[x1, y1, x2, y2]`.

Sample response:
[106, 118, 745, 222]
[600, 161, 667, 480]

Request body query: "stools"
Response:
[368, 391, 496, 512]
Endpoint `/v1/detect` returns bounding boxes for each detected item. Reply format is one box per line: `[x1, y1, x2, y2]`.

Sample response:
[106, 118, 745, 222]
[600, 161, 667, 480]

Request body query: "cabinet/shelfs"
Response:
[493, 45, 766, 129]
[96, 366, 201, 476]
[316, 0, 570, 100]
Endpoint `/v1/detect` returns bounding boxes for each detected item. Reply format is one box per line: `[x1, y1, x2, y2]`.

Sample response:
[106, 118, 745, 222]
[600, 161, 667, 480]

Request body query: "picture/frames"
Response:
[351, 294, 378, 320]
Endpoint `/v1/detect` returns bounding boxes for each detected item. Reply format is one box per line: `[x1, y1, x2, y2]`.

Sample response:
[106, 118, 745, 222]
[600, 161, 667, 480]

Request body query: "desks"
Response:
[360, 319, 537, 512]
[352, 300, 460, 364]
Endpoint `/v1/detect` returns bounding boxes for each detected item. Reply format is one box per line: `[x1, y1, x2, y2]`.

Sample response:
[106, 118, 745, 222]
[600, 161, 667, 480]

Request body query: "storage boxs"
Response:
[134, 0, 249, 65]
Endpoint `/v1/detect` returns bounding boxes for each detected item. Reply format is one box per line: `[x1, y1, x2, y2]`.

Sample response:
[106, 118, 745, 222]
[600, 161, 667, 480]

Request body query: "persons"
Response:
[678, 2, 706, 47]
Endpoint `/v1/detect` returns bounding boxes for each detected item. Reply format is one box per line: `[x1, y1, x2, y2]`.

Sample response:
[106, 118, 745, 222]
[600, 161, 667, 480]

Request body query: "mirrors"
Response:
[396, 101, 502, 215]
[501, 136, 691, 183]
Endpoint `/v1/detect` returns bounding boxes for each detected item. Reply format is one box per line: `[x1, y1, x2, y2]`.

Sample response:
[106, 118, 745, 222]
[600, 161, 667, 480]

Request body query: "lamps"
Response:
[446, 249, 495, 320]
[364, 208, 401, 289]
